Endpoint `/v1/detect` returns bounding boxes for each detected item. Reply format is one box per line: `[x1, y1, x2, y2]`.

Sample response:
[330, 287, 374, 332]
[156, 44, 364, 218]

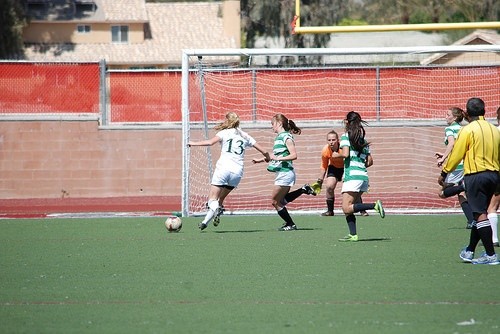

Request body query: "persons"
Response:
[331, 110, 385, 241]
[434, 97, 500, 264]
[317, 129, 369, 216]
[251, 113, 317, 231]
[186, 111, 271, 230]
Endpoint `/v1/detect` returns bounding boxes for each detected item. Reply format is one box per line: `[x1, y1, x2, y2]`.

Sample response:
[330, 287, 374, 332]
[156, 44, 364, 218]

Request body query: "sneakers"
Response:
[470, 253, 500, 266]
[466, 220, 476, 228]
[360, 210, 369, 216]
[302, 183, 316, 196]
[338, 233, 359, 242]
[374, 199, 385, 218]
[459, 246, 474, 262]
[279, 223, 296, 231]
[492, 242, 499, 247]
[213, 208, 223, 227]
[198, 222, 207, 230]
[321, 210, 334, 216]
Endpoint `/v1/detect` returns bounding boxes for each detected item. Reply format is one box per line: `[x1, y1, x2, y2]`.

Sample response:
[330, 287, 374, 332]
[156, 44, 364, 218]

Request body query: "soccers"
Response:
[165, 215, 183, 232]
